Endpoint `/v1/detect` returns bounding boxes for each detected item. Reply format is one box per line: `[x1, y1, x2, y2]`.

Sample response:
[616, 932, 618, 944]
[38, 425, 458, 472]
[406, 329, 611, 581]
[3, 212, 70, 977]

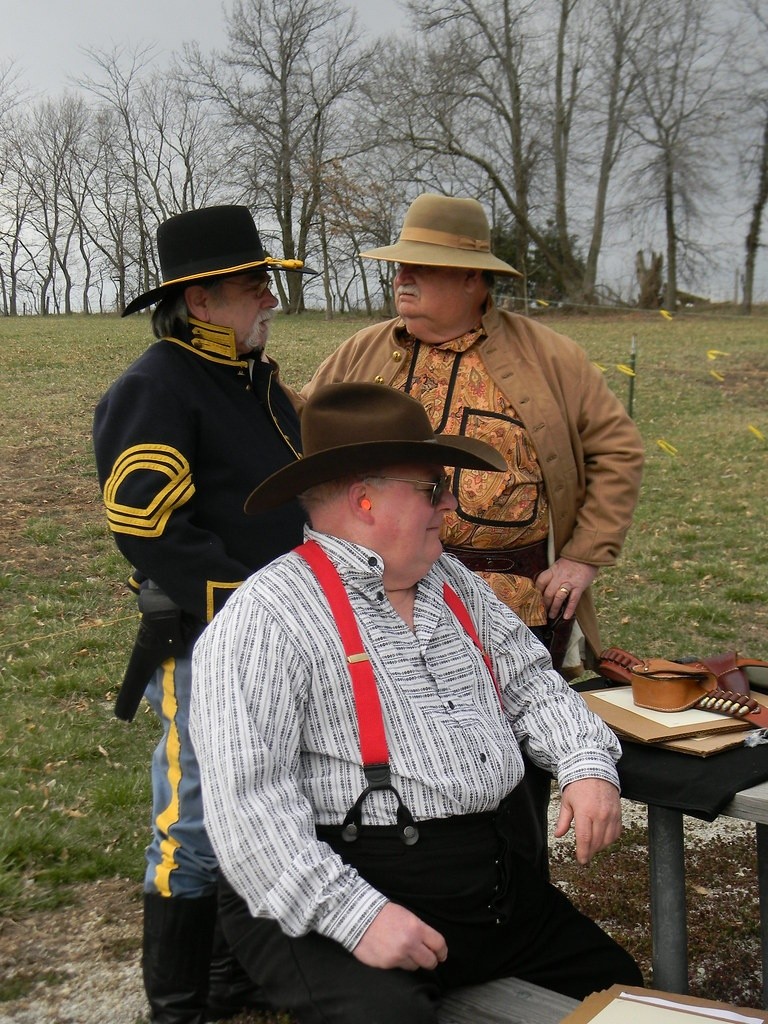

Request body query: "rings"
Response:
[560, 587, 571, 596]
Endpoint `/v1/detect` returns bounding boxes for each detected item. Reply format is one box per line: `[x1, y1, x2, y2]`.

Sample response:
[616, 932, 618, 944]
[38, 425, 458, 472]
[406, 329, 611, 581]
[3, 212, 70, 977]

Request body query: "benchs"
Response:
[434, 975, 583, 1023]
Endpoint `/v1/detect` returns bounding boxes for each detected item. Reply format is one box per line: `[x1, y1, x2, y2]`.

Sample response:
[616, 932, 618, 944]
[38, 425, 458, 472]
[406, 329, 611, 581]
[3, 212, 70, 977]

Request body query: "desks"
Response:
[646, 781, 768, 997]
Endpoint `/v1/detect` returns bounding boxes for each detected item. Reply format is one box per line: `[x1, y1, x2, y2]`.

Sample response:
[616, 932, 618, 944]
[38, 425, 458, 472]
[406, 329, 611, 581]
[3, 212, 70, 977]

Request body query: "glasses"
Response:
[225, 280, 274, 298]
[365, 474, 452, 508]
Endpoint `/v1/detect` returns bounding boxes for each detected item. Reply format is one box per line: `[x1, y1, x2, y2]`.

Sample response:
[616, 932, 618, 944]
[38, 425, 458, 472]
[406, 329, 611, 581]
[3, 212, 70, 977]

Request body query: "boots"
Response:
[141, 892, 215, 1024]
[207, 898, 284, 1018]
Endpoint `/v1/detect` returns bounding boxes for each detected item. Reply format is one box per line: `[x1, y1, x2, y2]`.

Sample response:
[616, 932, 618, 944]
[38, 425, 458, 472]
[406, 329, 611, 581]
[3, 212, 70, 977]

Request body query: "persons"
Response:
[302, 194, 644, 863]
[89, 202, 304, 1024]
[186, 383, 644, 1024]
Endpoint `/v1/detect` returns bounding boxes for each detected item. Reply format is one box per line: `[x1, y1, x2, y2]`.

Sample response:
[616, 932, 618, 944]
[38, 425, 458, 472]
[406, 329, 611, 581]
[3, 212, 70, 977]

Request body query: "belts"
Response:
[442, 538, 549, 584]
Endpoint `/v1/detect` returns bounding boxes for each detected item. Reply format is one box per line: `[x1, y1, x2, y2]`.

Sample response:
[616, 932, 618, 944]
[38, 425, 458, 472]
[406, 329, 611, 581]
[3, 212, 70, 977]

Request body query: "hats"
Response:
[358, 193, 524, 279]
[243, 381, 509, 516]
[118, 204, 318, 318]
